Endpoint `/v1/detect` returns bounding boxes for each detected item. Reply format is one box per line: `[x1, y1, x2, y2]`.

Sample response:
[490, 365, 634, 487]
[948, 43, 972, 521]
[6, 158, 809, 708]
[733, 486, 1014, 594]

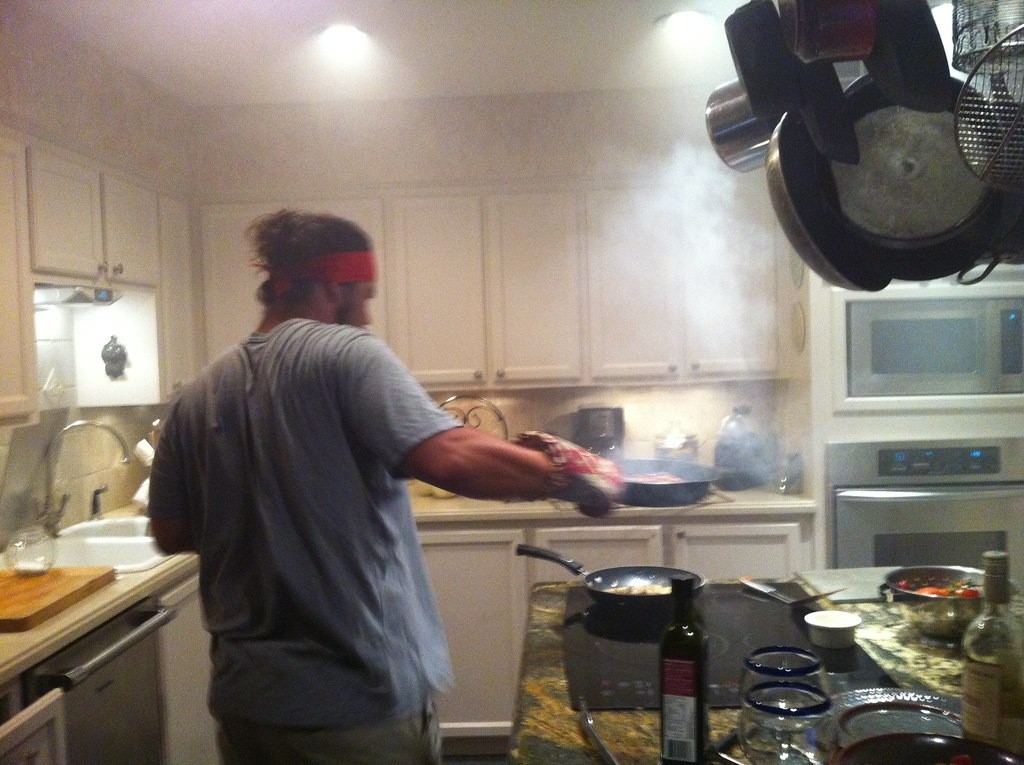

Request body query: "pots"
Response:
[516, 545, 705, 616]
[611, 458, 724, 506]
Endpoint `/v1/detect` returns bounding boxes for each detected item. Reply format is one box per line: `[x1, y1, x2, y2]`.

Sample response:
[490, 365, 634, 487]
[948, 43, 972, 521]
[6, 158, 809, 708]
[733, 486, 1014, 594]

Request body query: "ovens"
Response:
[824, 436, 1024, 588]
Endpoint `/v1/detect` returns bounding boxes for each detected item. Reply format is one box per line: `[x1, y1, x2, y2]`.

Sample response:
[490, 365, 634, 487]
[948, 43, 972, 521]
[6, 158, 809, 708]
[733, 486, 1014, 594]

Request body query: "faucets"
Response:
[35, 420, 132, 539]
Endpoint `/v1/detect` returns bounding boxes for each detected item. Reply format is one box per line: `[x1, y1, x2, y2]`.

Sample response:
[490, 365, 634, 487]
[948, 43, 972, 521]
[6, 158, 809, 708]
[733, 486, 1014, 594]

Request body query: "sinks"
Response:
[39, 540, 176, 574]
[74, 518, 154, 539]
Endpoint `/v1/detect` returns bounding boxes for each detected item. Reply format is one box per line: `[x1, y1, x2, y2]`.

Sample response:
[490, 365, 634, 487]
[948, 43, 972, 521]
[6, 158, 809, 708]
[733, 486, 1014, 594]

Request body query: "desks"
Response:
[508, 566, 1024, 765]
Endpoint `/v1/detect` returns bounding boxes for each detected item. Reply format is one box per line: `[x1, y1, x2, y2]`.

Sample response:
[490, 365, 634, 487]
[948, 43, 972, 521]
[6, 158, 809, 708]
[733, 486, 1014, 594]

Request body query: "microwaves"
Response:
[848, 303, 1024, 398]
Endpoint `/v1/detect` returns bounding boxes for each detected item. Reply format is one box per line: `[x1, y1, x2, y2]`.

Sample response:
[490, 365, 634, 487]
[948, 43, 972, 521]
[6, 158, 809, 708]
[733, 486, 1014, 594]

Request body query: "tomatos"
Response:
[898, 580, 981, 598]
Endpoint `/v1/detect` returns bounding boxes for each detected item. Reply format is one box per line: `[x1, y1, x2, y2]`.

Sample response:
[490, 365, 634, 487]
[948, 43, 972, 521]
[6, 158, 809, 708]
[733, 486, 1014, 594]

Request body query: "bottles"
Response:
[659, 575, 711, 765]
[963, 550, 1024, 756]
[713, 405, 763, 490]
[6, 525, 56, 574]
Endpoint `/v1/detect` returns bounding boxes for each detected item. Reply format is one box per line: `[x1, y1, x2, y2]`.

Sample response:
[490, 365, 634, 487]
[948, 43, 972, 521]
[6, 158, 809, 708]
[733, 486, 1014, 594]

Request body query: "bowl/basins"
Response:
[834, 733, 1021, 765]
[882, 566, 1019, 641]
[804, 610, 863, 649]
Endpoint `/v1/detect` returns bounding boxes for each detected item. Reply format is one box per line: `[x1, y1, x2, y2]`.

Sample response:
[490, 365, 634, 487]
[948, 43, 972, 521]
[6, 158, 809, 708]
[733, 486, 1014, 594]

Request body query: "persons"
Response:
[147, 206, 623, 765]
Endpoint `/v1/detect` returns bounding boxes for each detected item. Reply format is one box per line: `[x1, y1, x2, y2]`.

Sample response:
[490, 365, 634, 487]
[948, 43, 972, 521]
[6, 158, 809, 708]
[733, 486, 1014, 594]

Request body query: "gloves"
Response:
[512, 432, 626, 518]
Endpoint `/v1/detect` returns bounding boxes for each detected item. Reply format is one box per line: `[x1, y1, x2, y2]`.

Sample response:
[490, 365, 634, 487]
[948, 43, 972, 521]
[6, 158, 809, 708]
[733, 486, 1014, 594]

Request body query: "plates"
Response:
[812, 688, 961, 753]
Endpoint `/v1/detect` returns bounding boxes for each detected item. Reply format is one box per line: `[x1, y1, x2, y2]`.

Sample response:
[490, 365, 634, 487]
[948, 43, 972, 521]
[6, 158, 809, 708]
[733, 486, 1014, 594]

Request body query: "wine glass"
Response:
[738, 681, 834, 765]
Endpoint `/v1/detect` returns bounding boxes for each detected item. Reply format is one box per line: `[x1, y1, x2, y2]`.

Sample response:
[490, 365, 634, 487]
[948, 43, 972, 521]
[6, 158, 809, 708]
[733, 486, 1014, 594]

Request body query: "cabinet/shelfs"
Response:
[387, 183, 585, 394]
[409, 528, 527, 738]
[25, 132, 161, 294]
[0, 125, 42, 429]
[531, 521, 821, 594]
[585, 174, 781, 386]
[75, 189, 202, 409]
[196, 195, 388, 403]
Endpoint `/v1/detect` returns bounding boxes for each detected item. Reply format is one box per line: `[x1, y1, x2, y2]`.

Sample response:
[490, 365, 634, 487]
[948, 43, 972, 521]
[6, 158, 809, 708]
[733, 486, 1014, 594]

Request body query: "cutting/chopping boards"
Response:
[0, 567, 116, 632]
[794, 566, 908, 603]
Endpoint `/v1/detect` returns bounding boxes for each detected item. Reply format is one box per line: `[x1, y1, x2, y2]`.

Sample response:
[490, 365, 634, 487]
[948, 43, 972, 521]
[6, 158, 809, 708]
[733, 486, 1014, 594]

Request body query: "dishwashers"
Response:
[22, 595, 181, 765]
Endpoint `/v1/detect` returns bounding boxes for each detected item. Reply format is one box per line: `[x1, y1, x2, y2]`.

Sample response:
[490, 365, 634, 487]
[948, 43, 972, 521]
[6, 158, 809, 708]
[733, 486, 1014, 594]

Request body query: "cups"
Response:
[738, 645, 828, 703]
